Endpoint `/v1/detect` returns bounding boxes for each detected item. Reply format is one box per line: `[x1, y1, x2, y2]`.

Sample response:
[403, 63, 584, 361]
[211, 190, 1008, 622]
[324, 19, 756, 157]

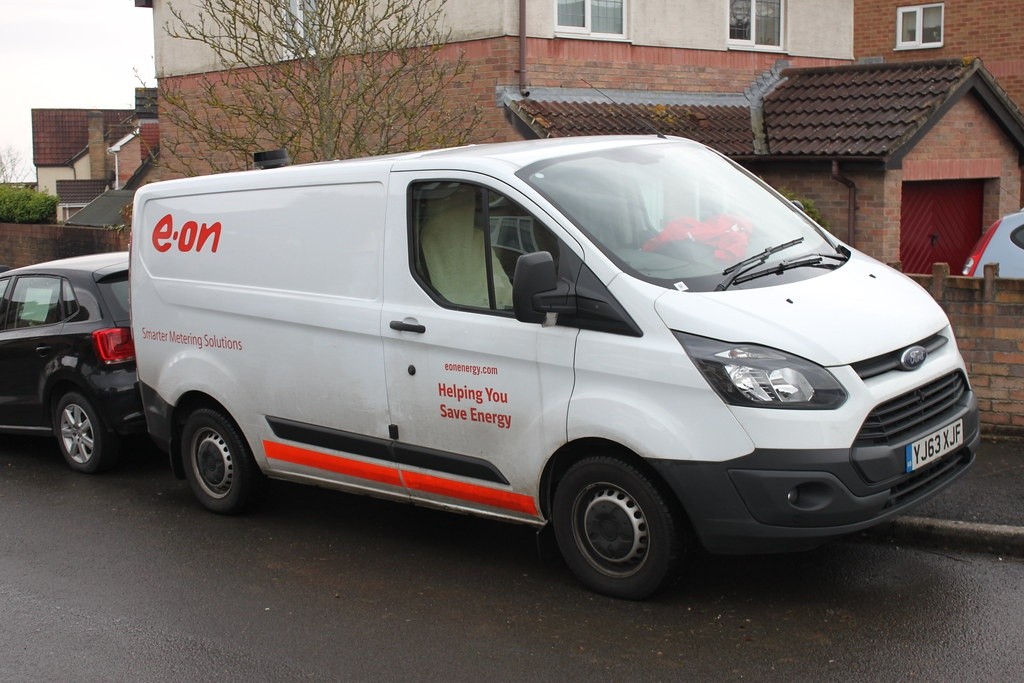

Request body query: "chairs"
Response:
[420, 190, 514, 307]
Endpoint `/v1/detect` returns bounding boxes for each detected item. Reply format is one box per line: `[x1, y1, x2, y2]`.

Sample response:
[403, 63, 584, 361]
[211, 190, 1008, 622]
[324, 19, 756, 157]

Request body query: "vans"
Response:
[129, 132, 986, 597]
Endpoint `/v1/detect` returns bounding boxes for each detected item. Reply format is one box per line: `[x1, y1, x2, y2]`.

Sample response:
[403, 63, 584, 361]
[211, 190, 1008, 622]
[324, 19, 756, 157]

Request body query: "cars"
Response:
[963, 210, 1024, 280]
[0, 249, 147, 474]
